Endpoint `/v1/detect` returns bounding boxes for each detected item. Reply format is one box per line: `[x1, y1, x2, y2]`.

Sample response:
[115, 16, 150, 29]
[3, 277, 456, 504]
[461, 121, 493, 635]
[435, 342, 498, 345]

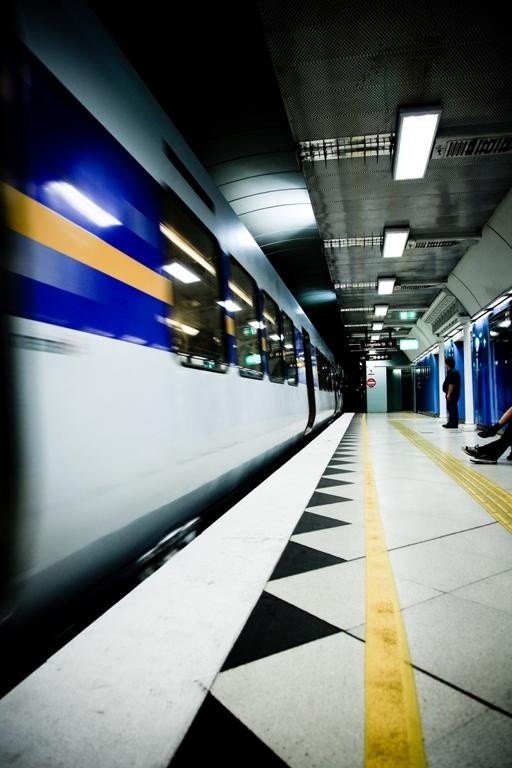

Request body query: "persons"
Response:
[442, 358, 460, 429]
[464, 407, 512, 464]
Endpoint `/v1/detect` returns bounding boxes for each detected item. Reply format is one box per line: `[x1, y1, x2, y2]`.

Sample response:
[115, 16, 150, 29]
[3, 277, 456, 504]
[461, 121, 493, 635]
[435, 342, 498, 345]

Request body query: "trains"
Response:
[0, 1, 348, 631]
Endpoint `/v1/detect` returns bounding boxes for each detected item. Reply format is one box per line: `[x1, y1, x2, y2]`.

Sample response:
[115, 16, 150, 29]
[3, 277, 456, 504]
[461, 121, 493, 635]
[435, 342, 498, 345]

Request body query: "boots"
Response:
[478, 422, 501, 438]
[507, 452, 512, 460]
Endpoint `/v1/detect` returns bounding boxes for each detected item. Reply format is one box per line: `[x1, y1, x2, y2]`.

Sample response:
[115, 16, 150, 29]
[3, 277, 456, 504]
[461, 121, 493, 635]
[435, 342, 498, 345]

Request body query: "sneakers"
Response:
[442, 424, 458, 428]
[461, 443, 497, 463]
